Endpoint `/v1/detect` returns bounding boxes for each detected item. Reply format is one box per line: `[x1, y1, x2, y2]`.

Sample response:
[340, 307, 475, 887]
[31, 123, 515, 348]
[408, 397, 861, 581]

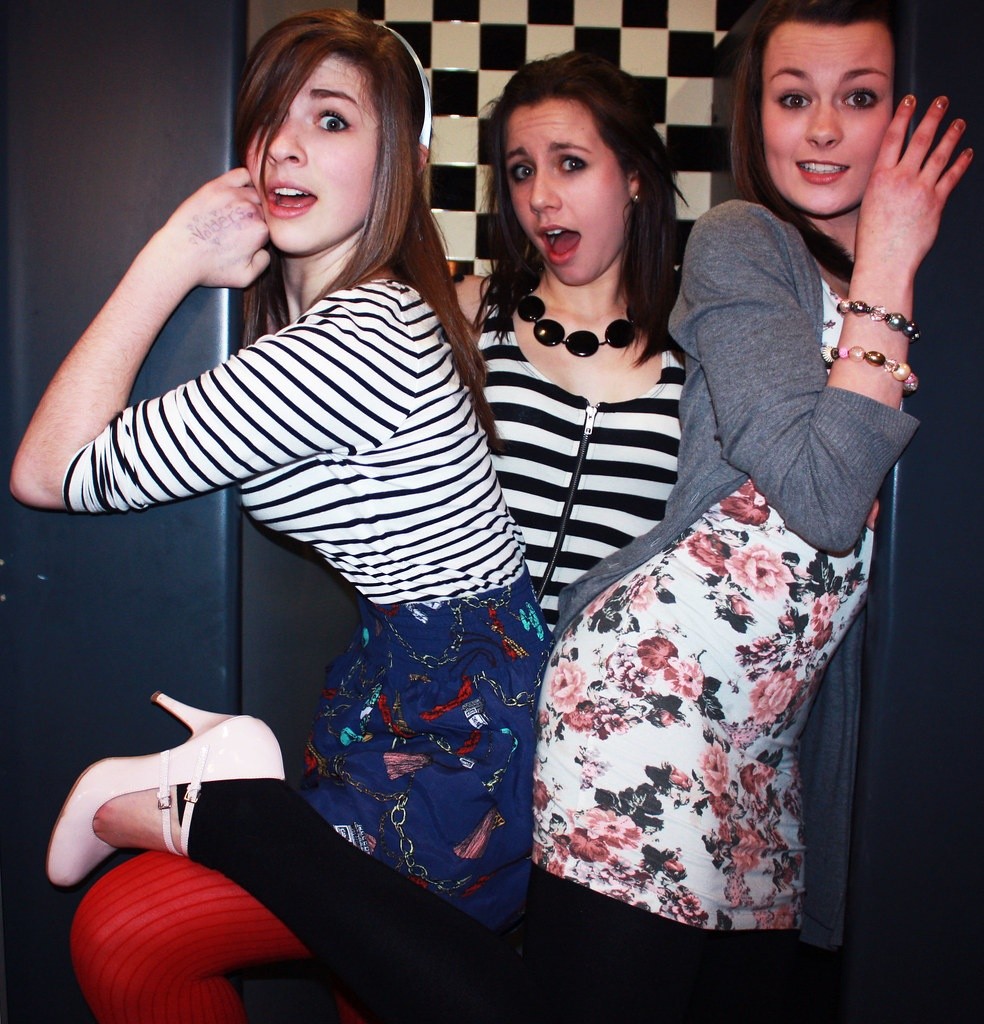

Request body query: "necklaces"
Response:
[509, 269, 641, 358]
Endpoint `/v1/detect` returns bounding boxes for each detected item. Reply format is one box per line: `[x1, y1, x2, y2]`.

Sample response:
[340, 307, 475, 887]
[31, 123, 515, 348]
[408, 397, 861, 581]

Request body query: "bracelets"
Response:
[835, 298, 922, 342]
[829, 344, 919, 398]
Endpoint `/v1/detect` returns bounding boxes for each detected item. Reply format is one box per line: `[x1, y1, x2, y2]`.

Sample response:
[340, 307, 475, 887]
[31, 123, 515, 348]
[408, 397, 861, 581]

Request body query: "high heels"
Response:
[47, 690, 286, 887]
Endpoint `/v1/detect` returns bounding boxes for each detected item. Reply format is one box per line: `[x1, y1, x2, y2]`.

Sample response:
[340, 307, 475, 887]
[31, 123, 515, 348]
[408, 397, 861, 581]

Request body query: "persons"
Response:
[444, 49, 689, 647]
[10, 3, 556, 1022]
[46, 0, 973, 1024]
